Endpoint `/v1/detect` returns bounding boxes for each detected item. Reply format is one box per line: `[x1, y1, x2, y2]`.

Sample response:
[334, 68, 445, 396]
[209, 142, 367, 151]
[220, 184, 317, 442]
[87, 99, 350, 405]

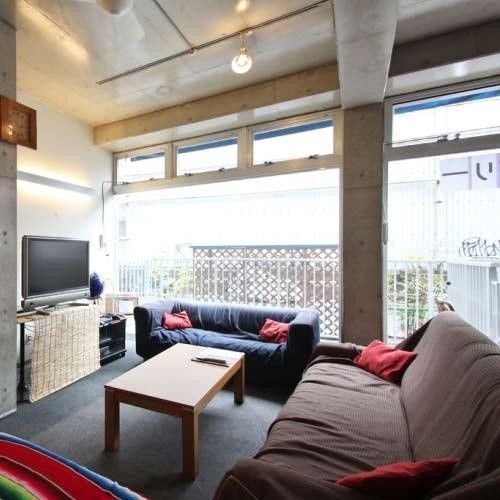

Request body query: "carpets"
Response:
[32, 390, 286, 499]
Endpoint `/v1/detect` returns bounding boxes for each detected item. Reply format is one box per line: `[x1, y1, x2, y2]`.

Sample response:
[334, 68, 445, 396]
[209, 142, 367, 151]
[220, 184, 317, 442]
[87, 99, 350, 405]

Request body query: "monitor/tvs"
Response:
[21, 234, 90, 315]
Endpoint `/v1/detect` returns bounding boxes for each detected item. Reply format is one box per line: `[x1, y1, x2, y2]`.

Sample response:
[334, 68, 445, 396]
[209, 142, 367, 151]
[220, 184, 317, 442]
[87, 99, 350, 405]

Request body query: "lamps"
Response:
[229, 34, 253, 75]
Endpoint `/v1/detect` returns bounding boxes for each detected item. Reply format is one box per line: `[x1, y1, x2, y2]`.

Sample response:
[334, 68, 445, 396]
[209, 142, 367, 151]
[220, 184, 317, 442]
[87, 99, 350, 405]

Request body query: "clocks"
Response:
[0, 95, 37, 149]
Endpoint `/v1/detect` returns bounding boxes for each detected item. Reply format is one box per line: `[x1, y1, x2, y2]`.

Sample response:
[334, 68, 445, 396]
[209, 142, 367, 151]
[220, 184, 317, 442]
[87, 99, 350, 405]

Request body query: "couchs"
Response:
[133, 301, 320, 386]
[214, 313, 498, 499]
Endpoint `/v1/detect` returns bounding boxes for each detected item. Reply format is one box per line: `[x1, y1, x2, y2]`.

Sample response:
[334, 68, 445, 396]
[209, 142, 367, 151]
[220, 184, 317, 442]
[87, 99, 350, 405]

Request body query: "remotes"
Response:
[196, 356, 227, 363]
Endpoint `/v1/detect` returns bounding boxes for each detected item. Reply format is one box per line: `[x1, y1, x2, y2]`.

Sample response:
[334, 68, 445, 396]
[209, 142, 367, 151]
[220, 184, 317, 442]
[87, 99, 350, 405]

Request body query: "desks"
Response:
[106, 295, 140, 315]
[17, 303, 94, 403]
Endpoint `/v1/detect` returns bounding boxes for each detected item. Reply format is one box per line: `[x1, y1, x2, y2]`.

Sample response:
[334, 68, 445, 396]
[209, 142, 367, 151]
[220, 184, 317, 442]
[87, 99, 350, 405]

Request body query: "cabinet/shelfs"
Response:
[101, 314, 127, 364]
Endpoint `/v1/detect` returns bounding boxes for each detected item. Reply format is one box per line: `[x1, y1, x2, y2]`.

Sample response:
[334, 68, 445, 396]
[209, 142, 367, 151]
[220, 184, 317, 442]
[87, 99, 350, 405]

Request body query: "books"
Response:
[191, 352, 238, 367]
[196, 350, 235, 365]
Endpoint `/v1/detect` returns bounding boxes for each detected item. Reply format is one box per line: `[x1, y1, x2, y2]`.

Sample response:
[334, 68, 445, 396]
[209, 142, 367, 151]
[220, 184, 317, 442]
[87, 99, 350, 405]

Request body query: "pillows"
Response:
[162, 310, 193, 329]
[352, 339, 417, 385]
[260, 318, 291, 343]
[337, 458, 459, 500]
